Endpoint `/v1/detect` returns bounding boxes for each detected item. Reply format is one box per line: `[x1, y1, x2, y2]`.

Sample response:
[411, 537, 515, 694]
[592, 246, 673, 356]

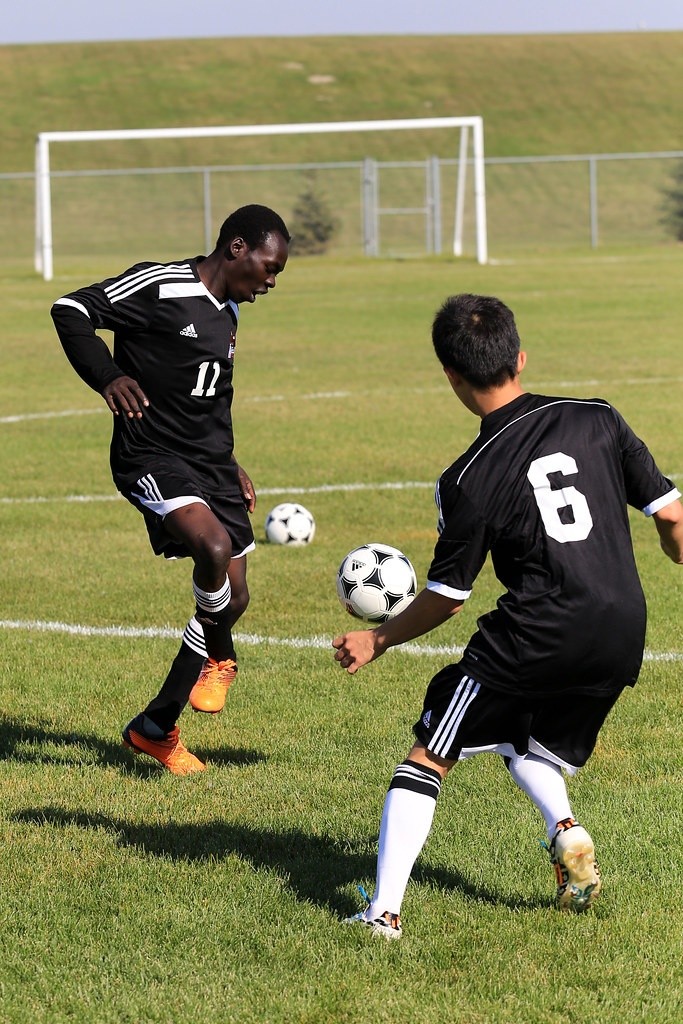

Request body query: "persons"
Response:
[328, 291, 682, 934]
[46, 204, 293, 774]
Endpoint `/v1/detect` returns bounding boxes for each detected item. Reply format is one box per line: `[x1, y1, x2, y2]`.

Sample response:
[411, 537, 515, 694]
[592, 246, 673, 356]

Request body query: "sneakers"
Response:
[342, 911, 403, 941]
[549, 818, 600, 914]
[188, 651, 237, 714]
[121, 723, 203, 773]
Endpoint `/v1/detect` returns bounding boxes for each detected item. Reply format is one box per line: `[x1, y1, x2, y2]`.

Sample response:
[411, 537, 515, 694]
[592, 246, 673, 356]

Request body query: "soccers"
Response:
[265, 502, 316, 545]
[335, 542, 418, 624]
